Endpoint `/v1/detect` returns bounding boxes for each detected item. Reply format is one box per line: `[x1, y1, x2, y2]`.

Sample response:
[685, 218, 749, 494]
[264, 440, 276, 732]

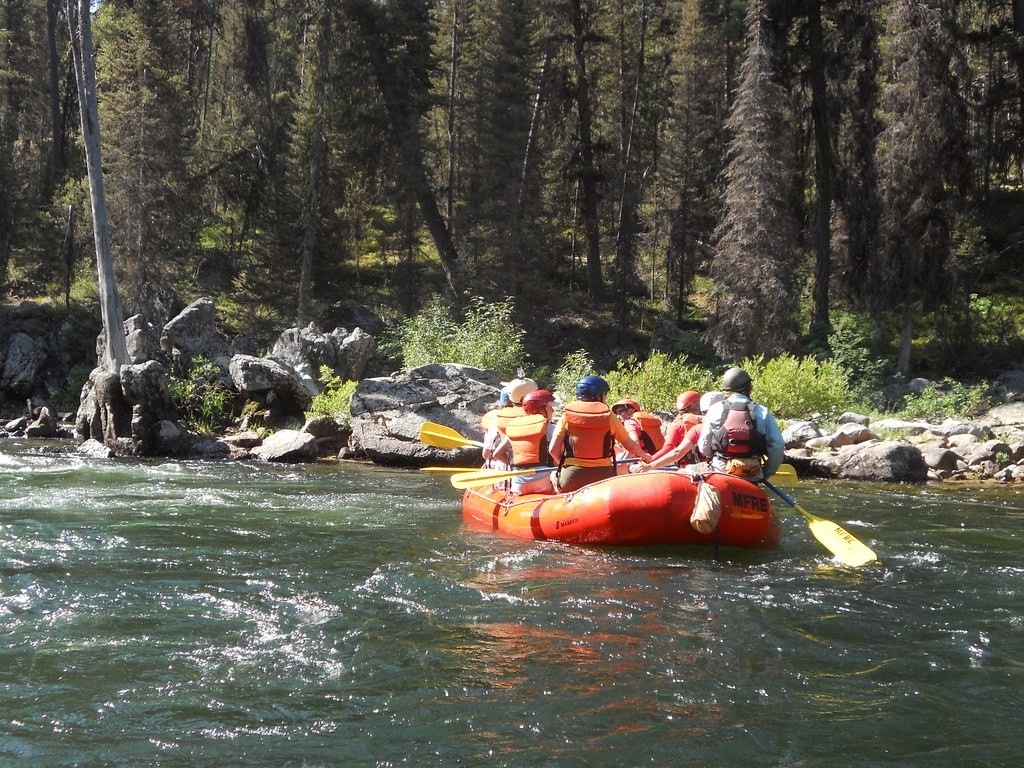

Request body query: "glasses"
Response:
[617, 407, 631, 415]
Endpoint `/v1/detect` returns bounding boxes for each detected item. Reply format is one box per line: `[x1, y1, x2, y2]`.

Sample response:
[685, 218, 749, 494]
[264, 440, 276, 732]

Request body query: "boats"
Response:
[460, 444, 773, 546]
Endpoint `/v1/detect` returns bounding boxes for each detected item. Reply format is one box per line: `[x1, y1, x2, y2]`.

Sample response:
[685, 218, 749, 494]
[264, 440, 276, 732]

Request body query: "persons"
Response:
[679, 368, 785, 485]
[549, 374, 651, 493]
[629, 391, 726, 475]
[646, 390, 702, 469]
[494, 389, 563, 497]
[612, 399, 664, 475]
[481, 367, 538, 489]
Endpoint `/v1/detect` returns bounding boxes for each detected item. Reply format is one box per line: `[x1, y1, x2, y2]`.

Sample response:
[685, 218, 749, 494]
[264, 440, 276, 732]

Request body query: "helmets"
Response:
[509, 378, 538, 403]
[577, 376, 610, 399]
[612, 399, 640, 415]
[700, 391, 725, 411]
[500, 387, 512, 407]
[723, 368, 751, 389]
[523, 390, 556, 415]
[677, 391, 700, 410]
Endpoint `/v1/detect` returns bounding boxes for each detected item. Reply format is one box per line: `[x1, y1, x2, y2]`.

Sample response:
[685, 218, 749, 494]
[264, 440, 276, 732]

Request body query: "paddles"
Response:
[420, 457, 643, 489]
[421, 422, 484, 450]
[762, 480, 878, 567]
[654, 464, 798, 488]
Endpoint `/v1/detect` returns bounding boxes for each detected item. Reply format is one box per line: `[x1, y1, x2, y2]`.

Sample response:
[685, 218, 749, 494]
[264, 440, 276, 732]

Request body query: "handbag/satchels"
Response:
[726, 458, 760, 477]
